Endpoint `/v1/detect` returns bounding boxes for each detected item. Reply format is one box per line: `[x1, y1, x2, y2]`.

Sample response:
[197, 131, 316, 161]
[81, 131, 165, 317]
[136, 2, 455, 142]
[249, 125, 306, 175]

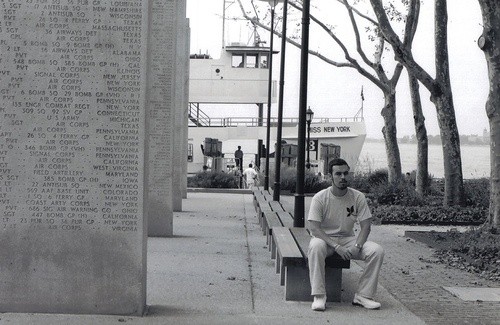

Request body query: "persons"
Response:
[307, 158, 383, 309]
[306, 160, 310, 171]
[235, 146, 243, 172]
[240, 164, 258, 189]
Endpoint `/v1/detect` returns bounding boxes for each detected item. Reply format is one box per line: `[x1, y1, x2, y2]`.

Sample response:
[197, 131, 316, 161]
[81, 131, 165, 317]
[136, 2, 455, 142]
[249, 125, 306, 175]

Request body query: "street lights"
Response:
[305, 106, 314, 171]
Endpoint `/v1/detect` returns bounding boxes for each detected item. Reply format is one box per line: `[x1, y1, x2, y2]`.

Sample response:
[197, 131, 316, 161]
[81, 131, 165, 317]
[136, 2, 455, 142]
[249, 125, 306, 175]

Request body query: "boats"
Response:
[187, 0, 367, 181]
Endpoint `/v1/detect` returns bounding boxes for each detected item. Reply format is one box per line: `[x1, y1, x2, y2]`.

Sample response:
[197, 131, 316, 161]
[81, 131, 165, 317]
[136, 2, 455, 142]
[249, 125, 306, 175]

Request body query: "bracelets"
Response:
[333, 243, 340, 251]
[353, 243, 363, 247]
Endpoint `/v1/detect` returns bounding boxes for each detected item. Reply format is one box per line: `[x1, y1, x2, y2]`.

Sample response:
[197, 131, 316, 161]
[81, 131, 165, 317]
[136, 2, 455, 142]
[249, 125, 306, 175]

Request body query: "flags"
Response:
[361, 85, 364, 101]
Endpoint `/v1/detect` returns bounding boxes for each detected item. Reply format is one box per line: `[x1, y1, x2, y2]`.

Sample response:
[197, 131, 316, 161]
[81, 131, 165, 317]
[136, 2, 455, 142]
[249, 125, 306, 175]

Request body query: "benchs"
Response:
[252, 186, 350, 303]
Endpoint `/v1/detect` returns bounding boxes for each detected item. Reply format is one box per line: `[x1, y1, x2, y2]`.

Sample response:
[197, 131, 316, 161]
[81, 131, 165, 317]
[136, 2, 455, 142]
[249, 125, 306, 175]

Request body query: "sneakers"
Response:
[352, 294, 380, 309]
[312, 294, 326, 311]
